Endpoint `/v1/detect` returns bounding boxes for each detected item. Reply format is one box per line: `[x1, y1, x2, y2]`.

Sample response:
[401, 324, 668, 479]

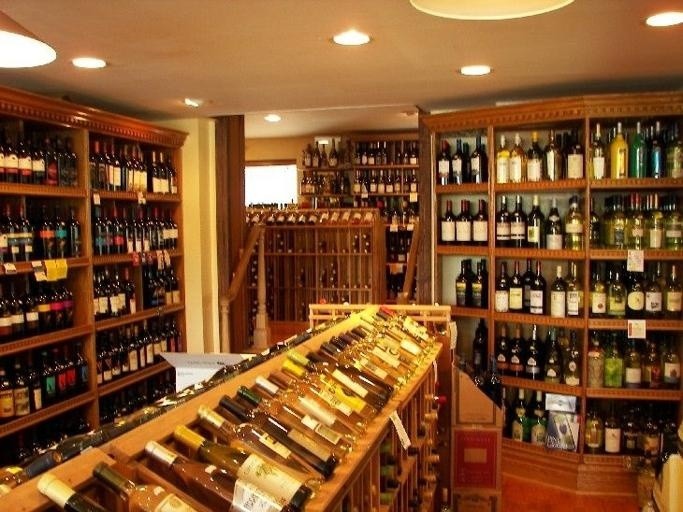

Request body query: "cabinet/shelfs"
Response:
[2, 305, 450, 504]
[492, 117, 587, 459]
[306, 137, 421, 306]
[431, 119, 496, 422]
[233, 209, 386, 328]
[588, 113, 683, 460]
[90, 133, 187, 422]
[1, 111, 83, 481]
